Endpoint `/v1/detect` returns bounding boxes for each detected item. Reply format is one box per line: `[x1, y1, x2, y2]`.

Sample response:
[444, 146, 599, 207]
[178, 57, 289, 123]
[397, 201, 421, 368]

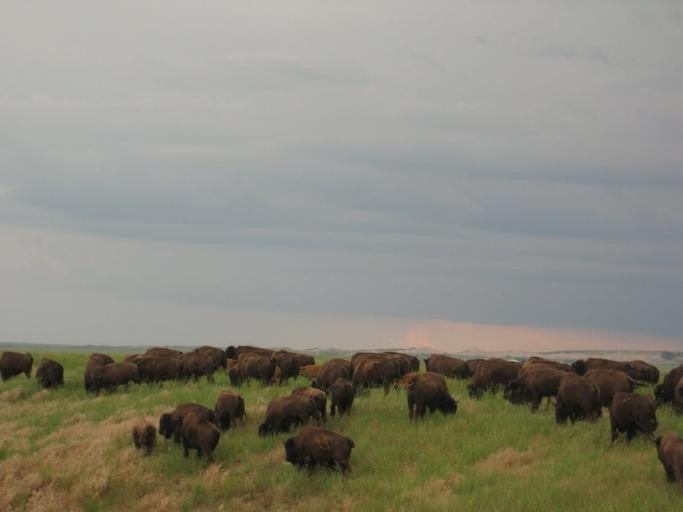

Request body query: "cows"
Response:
[656, 432, 683, 483]
[84, 345, 421, 394]
[424, 352, 683, 424]
[291, 387, 326, 426]
[406, 371, 460, 422]
[36, 358, 62, 388]
[283, 427, 355, 480]
[259, 395, 315, 438]
[610, 391, 658, 444]
[0, 349, 33, 379]
[133, 390, 249, 459]
[330, 377, 355, 419]
